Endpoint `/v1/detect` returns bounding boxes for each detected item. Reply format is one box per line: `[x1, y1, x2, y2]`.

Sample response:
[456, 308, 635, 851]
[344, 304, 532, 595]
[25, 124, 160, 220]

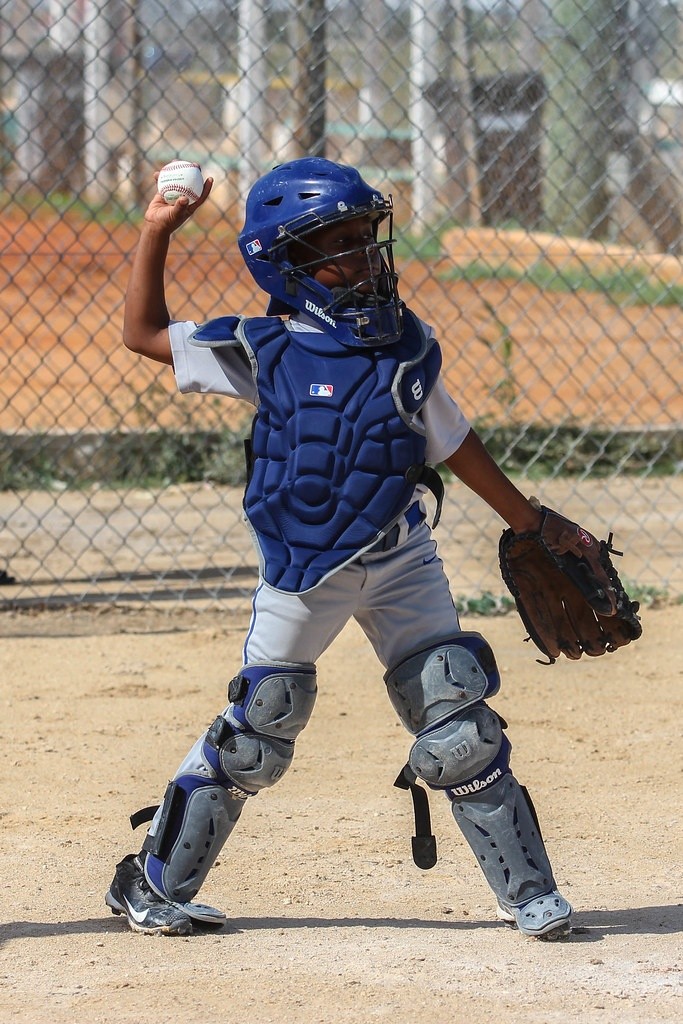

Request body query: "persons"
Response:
[104, 158, 643, 937]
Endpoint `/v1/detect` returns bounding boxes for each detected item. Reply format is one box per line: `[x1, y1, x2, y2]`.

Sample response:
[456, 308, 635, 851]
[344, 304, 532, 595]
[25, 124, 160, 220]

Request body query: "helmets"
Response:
[237, 158, 404, 348]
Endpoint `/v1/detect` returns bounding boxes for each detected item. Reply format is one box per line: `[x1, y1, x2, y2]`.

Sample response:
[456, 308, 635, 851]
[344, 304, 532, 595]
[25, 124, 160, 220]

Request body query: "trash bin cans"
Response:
[0, 49, 88, 195]
[427, 74, 542, 230]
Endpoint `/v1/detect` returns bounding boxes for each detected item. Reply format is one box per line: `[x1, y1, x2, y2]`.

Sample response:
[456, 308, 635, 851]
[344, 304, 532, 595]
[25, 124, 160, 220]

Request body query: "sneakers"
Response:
[496, 896, 572, 942]
[105, 854, 193, 937]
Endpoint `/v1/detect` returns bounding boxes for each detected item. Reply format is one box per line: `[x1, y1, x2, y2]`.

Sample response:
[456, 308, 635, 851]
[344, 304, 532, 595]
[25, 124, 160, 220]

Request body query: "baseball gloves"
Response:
[497, 505, 644, 666]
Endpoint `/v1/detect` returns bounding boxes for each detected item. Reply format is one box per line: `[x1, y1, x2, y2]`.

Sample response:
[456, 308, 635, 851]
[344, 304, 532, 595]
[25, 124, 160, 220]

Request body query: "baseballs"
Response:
[157, 157, 203, 204]
